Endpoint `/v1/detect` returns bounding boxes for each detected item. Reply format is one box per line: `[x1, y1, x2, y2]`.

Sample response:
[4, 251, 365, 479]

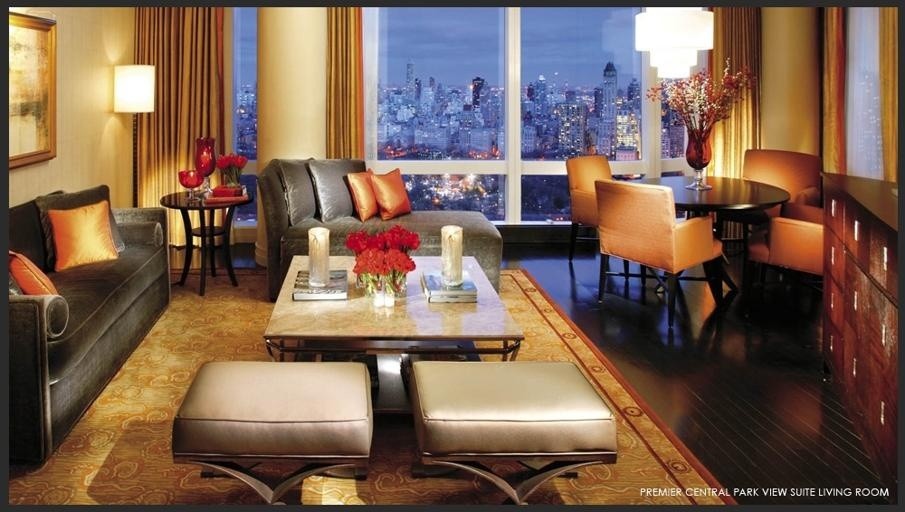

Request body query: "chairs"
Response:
[742, 201, 823, 317]
[595, 180, 722, 328]
[565, 155, 629, 281]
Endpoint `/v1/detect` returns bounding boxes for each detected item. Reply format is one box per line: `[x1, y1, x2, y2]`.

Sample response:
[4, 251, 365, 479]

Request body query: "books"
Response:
[420, 271, 478, 303]
[292, 269, 348, 301]
[204, 183, 249, 205]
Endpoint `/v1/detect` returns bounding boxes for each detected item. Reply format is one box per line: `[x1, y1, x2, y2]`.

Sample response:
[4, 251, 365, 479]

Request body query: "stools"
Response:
[173, 361, 374, 507]
[407, 361, 619, 505]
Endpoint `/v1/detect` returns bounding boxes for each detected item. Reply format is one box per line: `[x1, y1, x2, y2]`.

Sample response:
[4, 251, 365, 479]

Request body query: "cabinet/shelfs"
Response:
[823, 172, 898, 505]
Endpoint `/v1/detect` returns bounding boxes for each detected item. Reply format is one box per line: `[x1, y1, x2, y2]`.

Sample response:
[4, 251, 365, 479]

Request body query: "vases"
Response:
[686, 130, 712, 191]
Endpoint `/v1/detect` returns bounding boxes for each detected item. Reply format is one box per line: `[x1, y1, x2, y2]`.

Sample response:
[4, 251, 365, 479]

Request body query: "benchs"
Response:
[722, 149, 820, 251]
[258, 159, 502, 301]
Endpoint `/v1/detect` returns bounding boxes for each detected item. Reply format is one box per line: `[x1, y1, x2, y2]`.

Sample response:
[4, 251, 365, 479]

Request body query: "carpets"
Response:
[8, 267, 739, 505]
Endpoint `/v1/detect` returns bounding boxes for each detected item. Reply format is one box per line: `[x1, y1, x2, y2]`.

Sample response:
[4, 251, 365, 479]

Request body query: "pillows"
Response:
[49, 201, 118, 271]
[308, 158, 366, 222]
[35, 185, 125, 267]
[371, 168, 411, 221]
[347, 168, 379, 224]
[275, 159, 315, 225]
[8, 274, 24, 295]
[8, 250, 58, 296]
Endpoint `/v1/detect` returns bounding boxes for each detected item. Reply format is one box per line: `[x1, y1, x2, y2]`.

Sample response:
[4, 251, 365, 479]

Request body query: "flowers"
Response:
[646, 58, 757, 162]
[216, 153, 248, 186]
[345, 224, 421, 298]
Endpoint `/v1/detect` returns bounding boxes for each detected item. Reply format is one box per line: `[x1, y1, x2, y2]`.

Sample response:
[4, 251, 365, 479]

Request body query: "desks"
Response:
[160, 190, 253, 296]
[628, 177, 791, 320]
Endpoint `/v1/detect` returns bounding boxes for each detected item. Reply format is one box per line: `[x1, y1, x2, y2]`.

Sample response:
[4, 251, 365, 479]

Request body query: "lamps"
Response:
[114, 65, 155, 113]
[308, 227, 330, 287]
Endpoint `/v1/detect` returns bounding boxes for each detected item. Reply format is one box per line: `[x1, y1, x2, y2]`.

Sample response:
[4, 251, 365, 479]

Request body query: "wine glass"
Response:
[178, 171, 203, 202]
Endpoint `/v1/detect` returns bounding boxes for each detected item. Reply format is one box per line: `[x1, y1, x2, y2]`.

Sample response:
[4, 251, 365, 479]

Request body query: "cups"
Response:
[306, 226, 332, 288]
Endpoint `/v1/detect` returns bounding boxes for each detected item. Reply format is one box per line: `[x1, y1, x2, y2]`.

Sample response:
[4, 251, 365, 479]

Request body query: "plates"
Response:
[612, 172, 648, 180]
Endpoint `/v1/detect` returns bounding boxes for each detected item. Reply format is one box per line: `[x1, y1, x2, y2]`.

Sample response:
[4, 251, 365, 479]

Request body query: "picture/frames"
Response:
[8, 11, 57, 167]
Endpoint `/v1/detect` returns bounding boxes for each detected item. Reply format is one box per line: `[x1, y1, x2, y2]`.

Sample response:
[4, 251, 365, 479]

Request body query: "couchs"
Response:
[9, 191, 171, 466]
